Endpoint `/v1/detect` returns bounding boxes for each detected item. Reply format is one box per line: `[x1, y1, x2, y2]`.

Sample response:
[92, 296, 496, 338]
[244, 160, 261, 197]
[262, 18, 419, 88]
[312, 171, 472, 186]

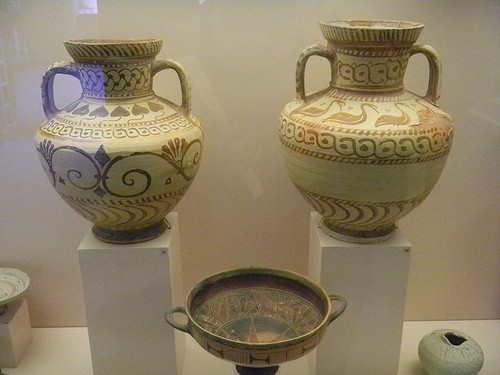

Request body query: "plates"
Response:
[0, 267, 31, 305]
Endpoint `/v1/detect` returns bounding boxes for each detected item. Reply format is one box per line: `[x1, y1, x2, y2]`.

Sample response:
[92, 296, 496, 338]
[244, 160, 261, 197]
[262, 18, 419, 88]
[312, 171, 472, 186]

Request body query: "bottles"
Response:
[418, 329, 484, 375]
[277, 18, 456, 245]
[33, 36, 205, 245]
